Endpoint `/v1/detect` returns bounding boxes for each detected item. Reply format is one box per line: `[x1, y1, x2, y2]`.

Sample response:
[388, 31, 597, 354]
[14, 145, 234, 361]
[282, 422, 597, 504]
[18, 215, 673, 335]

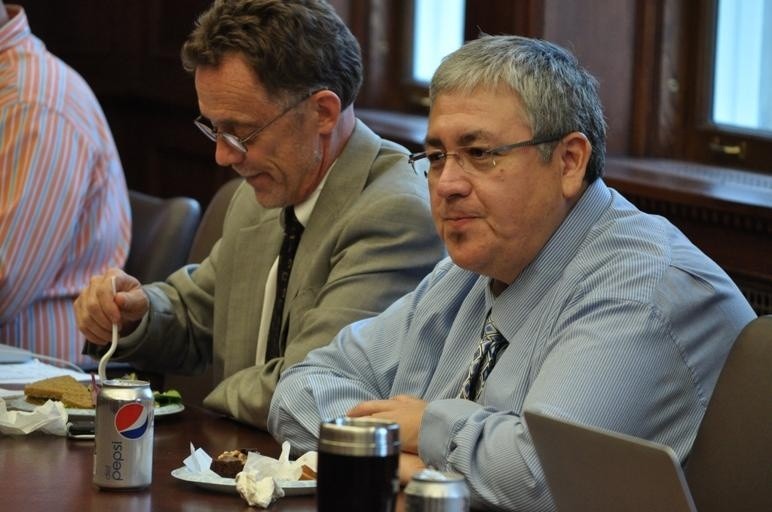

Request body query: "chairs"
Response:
[124, 174, 243, 407]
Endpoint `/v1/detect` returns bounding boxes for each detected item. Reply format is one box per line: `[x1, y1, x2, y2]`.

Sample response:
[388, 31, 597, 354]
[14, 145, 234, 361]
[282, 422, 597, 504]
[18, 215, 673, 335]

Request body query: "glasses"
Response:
[405, 134, 565, 179]
[193, 92, 322, 154]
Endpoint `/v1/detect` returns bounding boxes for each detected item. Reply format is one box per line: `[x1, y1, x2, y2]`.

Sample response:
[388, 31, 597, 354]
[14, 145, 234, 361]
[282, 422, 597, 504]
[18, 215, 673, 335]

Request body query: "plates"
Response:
[7, 375, 186, 419]
[169, 451, 318, 497]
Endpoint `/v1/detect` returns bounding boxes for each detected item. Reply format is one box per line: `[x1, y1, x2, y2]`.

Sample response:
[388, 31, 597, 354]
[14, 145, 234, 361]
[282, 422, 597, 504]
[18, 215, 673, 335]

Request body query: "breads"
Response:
[23, 375, 94, 409]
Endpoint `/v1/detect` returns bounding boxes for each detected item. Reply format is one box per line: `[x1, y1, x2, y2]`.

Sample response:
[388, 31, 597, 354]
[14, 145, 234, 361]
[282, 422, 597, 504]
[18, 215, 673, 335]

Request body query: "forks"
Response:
[97, 274, 120, 379]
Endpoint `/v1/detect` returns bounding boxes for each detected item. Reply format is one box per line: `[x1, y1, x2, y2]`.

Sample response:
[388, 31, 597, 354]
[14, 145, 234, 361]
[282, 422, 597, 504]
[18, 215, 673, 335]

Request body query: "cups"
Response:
[317, 416, 403, 511]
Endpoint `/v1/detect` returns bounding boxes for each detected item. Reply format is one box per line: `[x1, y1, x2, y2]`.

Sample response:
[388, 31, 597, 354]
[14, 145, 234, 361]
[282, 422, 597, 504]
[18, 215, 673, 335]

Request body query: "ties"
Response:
[456, 308, 505, 405]
[263, 203, 305, 362]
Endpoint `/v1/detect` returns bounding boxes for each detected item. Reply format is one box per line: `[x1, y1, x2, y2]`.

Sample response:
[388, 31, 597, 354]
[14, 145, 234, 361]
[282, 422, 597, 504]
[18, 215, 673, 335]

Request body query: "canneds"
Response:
[92, 379, 155, 492]
[403, 471, 471, 512]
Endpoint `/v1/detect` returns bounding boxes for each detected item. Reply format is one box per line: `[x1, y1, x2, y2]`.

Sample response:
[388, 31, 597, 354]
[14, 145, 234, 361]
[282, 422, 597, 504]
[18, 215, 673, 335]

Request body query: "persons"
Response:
[262, 31, 758, 510]
[73, 0, 446, 435]
[0, 2, 133, 370]
